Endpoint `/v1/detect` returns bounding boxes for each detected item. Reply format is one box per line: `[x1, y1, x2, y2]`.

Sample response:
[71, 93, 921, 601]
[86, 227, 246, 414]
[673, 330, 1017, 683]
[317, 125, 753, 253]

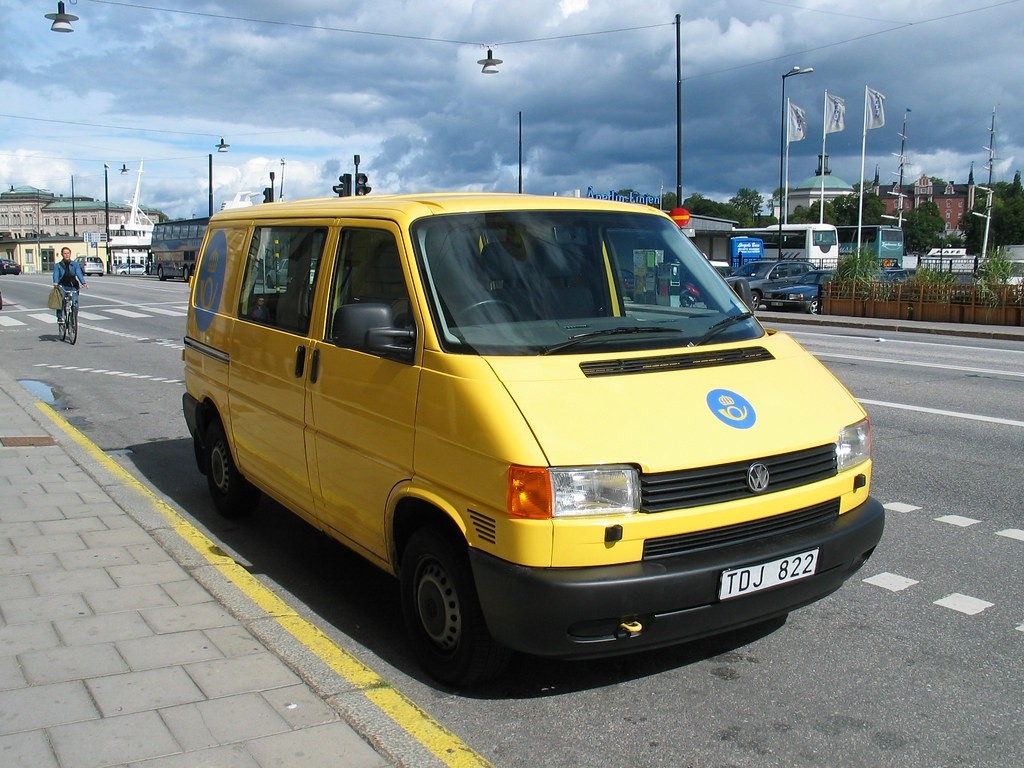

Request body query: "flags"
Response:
[824, 92, 845, 134]
[865, 87, 885, 129]
[788, 101, 807, 141]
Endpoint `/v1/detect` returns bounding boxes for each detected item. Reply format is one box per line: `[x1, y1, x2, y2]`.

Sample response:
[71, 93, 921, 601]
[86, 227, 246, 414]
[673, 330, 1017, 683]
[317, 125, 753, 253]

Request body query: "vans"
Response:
[183, 191, 887, 689]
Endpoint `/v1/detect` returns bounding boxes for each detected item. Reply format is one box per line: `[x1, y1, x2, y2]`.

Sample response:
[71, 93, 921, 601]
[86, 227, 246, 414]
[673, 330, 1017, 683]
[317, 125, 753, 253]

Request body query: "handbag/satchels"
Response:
[48, 286, 62, 310]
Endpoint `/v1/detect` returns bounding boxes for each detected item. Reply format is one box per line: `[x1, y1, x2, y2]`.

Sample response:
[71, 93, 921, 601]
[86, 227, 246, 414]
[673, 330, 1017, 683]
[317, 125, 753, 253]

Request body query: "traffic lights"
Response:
[333, 174, 351, 196]
[356, 173, 372, 195]
[262, 188, 273, 203]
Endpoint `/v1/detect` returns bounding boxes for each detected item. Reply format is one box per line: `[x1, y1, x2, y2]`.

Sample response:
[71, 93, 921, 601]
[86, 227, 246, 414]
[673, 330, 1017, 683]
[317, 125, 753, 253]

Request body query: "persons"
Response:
[53, 247, 89, 331]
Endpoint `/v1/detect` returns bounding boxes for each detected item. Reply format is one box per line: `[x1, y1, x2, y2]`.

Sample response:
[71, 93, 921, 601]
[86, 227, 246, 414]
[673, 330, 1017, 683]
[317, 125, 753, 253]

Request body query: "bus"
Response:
[151, 216, 211, 282]
[733, 224, 839, 271]
[835, 224, 904, 272]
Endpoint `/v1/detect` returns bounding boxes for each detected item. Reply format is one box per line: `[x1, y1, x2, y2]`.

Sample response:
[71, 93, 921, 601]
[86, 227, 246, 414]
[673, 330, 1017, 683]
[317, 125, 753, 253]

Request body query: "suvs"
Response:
[724, 260, 819, 311]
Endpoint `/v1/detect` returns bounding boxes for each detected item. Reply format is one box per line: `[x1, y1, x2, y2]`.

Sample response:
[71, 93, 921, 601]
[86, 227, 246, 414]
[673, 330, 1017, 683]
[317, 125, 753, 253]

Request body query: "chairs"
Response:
[536, 242, 598, 320]
[358, 240, 405, 308]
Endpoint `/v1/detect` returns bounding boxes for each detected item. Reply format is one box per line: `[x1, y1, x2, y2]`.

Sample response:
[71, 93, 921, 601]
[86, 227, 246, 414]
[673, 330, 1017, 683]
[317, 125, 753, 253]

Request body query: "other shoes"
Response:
[57, 317, 65, 323]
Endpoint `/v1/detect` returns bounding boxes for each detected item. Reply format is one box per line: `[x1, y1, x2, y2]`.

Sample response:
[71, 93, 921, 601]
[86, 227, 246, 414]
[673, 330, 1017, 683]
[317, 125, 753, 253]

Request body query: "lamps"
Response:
[44, 1, 79, 32]
[8, 185, 16, 193]
[215, 138, 230, 153]
[118, 163, 130, 175]
[477, 49, 503, 74]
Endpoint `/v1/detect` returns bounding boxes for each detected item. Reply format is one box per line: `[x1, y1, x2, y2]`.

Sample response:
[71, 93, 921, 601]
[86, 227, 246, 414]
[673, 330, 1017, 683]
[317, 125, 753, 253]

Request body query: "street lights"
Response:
[777, 66, 813, 260]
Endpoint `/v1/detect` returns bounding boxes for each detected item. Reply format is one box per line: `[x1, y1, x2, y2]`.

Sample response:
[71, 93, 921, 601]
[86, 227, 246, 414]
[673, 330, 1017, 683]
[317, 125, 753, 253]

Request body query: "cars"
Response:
[977, 259, 1024, 286]
[74, 256, 104, 276]
[760, 269, 836, 314]
[0, 258, 22, 276]
[116, 263, 146, 276]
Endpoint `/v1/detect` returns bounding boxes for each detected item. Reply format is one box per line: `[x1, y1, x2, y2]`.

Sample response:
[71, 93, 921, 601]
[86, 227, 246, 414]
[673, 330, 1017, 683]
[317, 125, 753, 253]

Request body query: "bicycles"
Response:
[53, 284, 86, 344]
